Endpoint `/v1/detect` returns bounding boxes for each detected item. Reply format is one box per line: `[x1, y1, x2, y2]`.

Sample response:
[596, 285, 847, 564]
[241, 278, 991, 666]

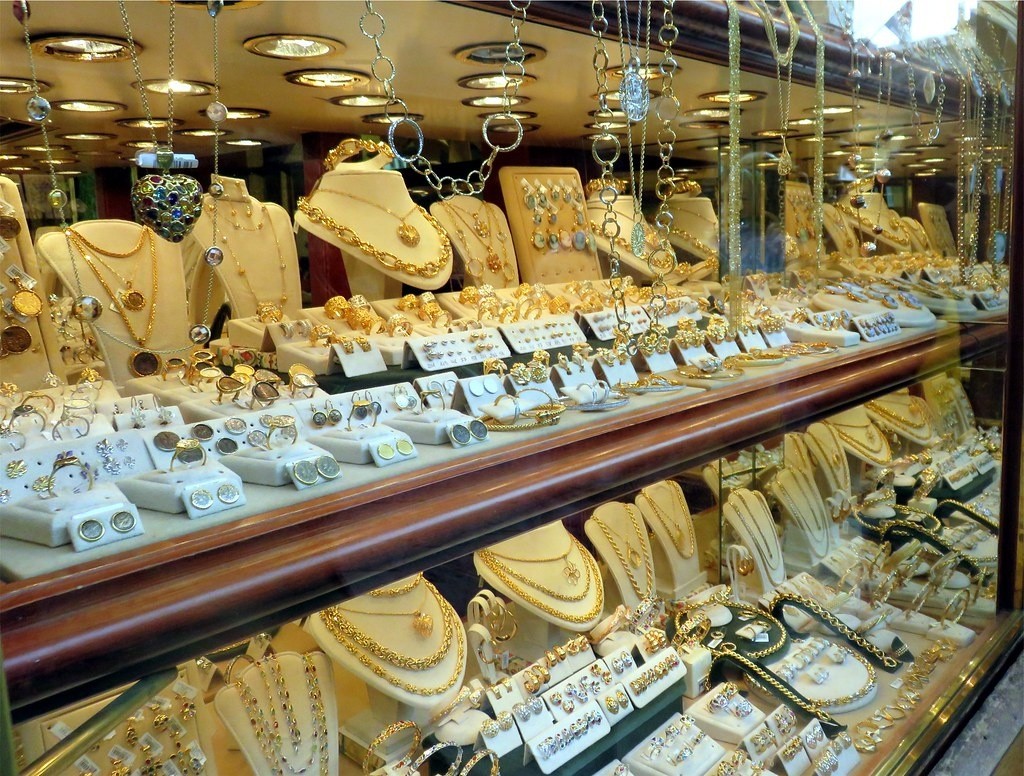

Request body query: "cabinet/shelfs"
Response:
[0, 2, 1024, 776]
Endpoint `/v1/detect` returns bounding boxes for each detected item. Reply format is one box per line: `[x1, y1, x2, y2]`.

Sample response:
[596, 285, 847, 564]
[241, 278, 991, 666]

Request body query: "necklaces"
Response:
[235, 480, 694, 776]
[713, 377, 1000, 587]
[0, 0, 1014, 356]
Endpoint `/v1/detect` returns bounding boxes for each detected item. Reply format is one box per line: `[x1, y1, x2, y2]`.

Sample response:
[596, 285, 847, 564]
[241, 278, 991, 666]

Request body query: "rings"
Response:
[482, 429, 999, 776]
[1, 248, 1009, 541]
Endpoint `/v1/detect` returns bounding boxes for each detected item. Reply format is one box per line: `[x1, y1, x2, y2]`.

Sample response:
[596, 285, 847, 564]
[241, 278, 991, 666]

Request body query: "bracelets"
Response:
[478, 405, 566, 431]
[782, 342, 840, 355]
[555, 391, 629, 410]
[726, 354, 787, 365]
[362, 469, 990, 776]
[612, 379, 687, 391]
[678, 364, 747, 378]
